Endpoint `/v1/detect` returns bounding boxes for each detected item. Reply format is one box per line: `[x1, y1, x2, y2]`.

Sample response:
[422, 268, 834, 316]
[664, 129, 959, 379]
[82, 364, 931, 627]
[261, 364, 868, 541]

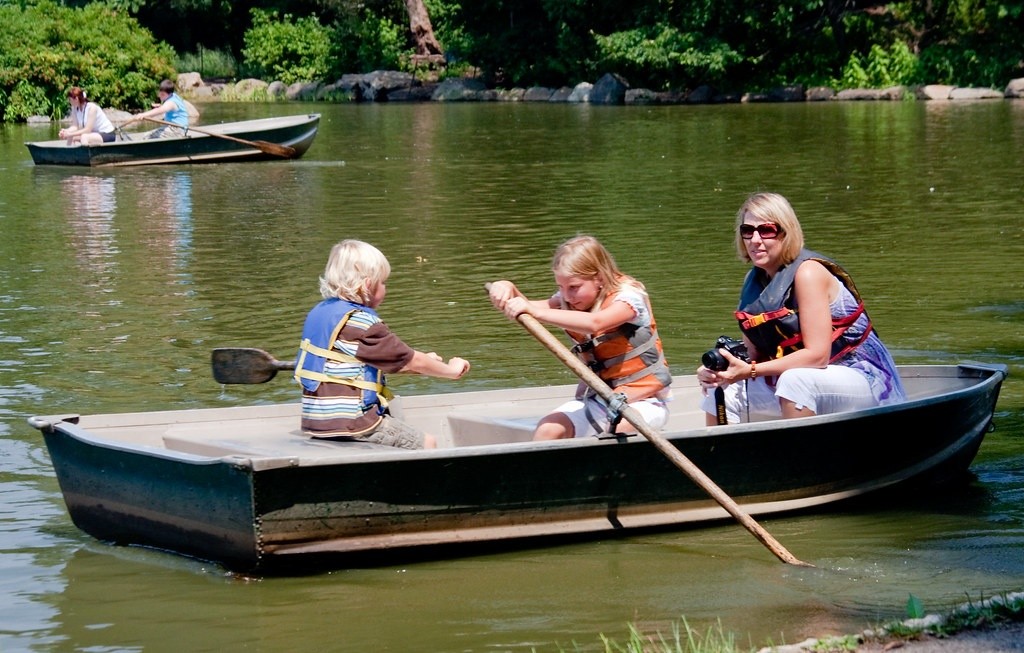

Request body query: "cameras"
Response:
[702, 335, 751, 372]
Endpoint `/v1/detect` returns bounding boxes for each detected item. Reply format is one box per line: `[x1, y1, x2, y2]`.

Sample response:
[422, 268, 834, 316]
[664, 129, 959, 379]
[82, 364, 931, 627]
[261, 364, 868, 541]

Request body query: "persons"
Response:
[295, 239, 471, 451]
[58, 87, 116, 146]
[696, 192, 907, 426]
[488, 235, 674, 441]
[136, 79, 188, 139]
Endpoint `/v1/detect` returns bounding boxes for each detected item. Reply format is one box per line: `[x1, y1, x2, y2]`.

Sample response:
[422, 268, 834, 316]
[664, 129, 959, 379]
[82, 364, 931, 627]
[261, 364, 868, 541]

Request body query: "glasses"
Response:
[740, 223, 783, 239]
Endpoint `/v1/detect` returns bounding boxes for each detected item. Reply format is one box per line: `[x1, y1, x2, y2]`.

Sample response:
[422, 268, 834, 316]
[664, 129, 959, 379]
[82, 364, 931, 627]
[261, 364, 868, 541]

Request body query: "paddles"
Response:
[482, 281, 815, 564]
[212, 347, 297, 384]
[115, 118, 134, 131]
[144, 117, 296, 158]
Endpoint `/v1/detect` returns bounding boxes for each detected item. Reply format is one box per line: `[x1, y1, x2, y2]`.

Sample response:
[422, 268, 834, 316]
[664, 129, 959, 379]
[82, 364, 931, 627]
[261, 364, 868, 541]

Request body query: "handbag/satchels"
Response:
[182, 99, 200, 124]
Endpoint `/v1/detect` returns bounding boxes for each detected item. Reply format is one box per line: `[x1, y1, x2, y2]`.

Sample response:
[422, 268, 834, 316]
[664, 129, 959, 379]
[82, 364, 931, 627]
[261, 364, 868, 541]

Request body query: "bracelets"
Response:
[750, 361, 757, 382]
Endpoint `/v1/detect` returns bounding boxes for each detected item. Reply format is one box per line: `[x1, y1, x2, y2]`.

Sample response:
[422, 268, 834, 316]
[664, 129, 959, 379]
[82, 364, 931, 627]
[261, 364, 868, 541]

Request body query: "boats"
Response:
[24, 112, 325, 170]
[27, 361, 1011, 579]
